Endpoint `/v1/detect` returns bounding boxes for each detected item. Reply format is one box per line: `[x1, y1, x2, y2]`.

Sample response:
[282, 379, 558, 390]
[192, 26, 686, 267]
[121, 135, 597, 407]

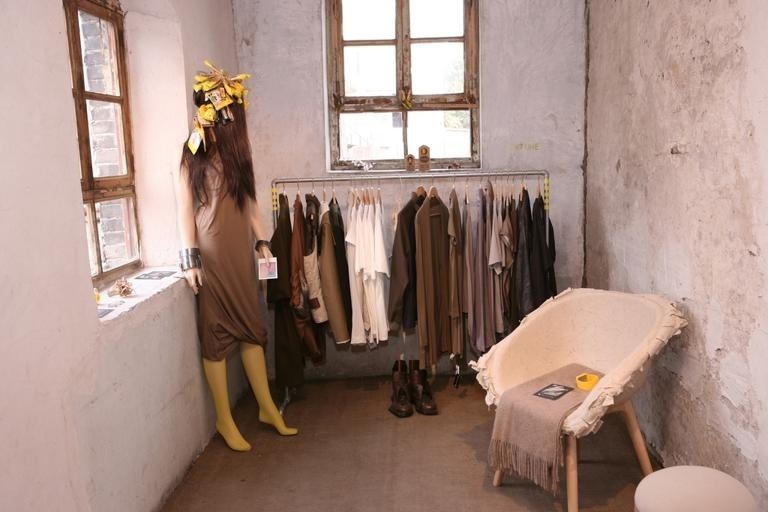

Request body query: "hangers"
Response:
[276, 171, 546, 226]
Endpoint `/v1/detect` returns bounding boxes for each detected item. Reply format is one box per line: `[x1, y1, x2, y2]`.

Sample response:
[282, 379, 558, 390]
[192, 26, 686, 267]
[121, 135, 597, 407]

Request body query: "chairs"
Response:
[475, 284, 690, 512]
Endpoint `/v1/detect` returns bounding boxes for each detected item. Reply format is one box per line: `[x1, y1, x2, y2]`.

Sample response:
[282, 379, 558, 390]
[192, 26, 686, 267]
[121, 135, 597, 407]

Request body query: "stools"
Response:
[633, 464, 757, 511]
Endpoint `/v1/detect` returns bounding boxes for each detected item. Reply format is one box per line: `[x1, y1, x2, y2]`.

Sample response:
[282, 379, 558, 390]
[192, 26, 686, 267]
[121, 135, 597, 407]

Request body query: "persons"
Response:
[177, 66, 298, 453]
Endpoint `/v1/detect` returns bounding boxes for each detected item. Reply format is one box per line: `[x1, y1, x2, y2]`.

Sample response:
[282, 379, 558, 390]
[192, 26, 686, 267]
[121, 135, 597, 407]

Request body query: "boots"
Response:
[410, 359, 438, 417]
[390, 360, 413, 417]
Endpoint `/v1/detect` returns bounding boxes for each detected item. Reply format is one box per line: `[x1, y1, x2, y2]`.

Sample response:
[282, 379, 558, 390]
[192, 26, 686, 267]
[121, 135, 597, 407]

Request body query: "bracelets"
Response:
[254, 239, 272, 250]
[179, 247, 202, 271]
